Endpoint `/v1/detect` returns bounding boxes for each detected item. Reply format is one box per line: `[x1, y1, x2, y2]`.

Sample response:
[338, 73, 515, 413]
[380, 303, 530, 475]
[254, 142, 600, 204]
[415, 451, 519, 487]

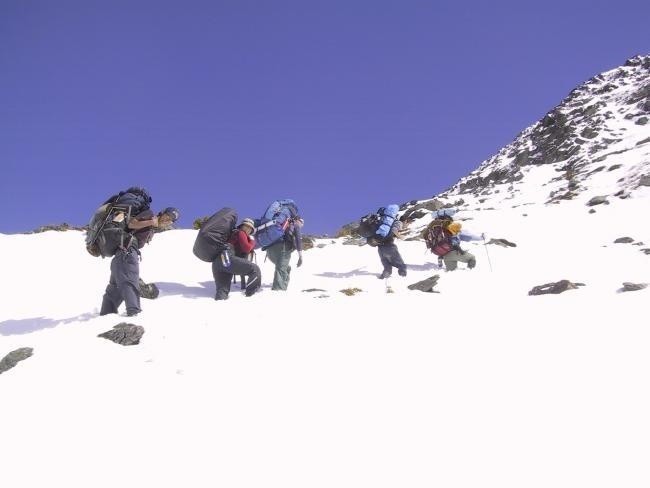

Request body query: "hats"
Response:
[166, 207, 178, 221]
[237, 218, 254, 233]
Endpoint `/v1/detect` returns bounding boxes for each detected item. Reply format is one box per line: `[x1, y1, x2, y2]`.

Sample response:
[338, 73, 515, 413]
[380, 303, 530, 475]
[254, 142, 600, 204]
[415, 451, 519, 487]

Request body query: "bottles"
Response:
[281, 216, 290, 231]
[221, 251, 232, 269]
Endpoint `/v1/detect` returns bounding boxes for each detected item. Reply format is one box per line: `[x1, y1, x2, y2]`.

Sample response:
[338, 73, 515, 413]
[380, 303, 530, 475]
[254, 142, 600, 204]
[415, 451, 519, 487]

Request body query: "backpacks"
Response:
[254, 199, 298, 247]
[358, 207, 394, 246]
[425, 225, 460, 255]
[85, 187, 152, 259]
[193, 207, 237, 261]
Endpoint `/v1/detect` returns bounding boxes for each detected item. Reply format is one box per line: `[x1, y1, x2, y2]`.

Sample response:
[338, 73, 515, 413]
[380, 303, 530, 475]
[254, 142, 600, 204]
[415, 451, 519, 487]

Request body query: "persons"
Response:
[100, 207, 180, 316]
[265, 218, 304, 289]
[435, 216, 486, 270]
[378, 207, 412, 278]
[213, 218, 261, 300]
